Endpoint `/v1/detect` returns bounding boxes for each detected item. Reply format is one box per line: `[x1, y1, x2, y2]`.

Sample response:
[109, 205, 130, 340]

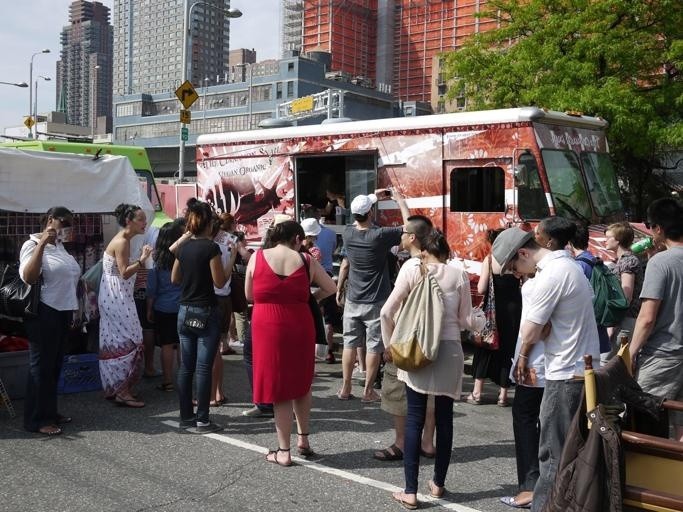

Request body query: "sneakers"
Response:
[197, 422, 222, 433]
[179, 419, 196, 429]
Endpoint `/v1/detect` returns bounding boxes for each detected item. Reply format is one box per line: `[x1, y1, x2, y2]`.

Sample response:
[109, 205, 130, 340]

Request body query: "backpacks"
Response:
[576, 255, 629, 328]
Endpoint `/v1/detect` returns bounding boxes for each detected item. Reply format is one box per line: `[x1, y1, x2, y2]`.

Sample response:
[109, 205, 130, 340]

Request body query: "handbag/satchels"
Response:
[0, 263, 40, 317]
[389, 273, 446, 372]
[465, 295, 500, 350]
[71, 260, 102, 329]
[232, 271, 248, 313]
[183, 311, 208, 330]
[310, 292, 327, 344]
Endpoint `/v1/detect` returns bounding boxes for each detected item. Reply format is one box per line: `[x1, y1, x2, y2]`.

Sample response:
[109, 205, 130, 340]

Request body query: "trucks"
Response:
[190, 106, 659, 368]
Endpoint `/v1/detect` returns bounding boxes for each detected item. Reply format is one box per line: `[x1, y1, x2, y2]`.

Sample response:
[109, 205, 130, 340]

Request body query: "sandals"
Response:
[297, 433, 313, 455]
[114, 395, 145, 407]
[266, 449, 291, 465]
[496, 391, 508, 407]
[240, 407, 274, 418]
[37, 424, 62, 435]
[157, 383, 173, 392]
[52, 414, 73, 423]
[462, 391, 482, 405]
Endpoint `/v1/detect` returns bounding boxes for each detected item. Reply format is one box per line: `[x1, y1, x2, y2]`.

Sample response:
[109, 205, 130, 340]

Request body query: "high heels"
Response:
[500, 496, 533, 507]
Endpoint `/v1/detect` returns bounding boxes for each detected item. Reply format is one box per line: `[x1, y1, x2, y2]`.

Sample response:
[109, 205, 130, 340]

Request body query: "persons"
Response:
[379, 228, 483, 511]
[488, 225, 603, 512]
[97, 190, 404, 434]
[498, 214, 577, 508]
[18, 206, 81, 436]
[461, 197, 682, 407]
[370, 214, 438, 461]
[244, 219, 338, 467]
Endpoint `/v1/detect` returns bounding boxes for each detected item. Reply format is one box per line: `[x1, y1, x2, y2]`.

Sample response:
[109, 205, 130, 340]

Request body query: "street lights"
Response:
[27, 48, 51, 139]
[177, 0, 249, 178]
[0, 79, 27, 90]
[33, 71, 51, 139]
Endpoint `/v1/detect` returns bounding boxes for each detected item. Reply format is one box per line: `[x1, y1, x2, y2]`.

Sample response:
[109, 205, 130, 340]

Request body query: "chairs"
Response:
[579, 335, 682, 512]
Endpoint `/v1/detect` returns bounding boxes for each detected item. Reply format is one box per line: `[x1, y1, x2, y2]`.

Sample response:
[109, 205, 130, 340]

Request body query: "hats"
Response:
[299, 218, 322, 236]
[351, 193, 379, 217]
[491, 227, 535, 276]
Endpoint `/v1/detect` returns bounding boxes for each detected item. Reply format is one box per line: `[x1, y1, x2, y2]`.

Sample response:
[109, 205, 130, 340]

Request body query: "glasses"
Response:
[400, 228, 416, 237]
[53, 216, 71, 227]
[644, 220, 652, 229]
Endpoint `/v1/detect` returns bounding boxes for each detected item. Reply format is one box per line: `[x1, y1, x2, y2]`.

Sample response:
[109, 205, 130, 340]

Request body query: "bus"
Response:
[3, 141, 177, 254]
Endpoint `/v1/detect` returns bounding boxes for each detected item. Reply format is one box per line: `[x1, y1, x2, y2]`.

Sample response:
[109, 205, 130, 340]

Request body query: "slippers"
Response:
[336, 389, 349, 400]
[428, 478, 444, 498]
[373, 444, 404, 461]
[361, 390, 382, 402]
[419, 446, 436, 459]
[392, 492, 419, 509]
[325, 353, 334, 363]
